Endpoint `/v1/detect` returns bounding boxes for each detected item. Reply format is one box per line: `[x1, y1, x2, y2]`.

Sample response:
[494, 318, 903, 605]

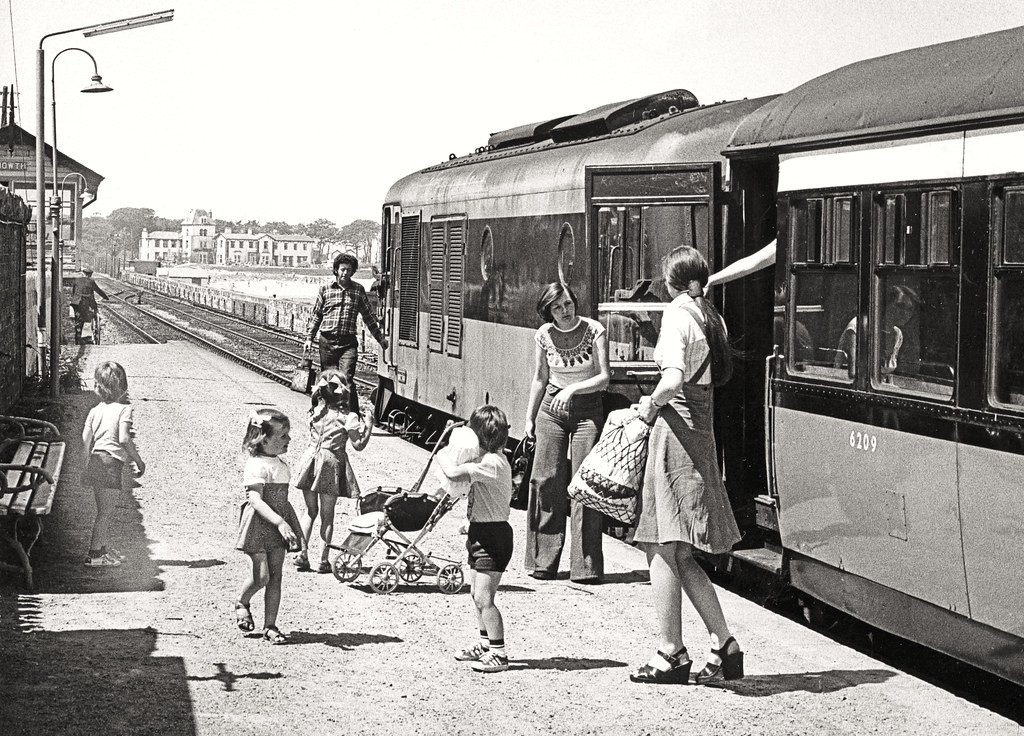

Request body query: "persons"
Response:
[78, 360, 145, 567]
[303, 253, 389, 414]
[232, 408, 297, 644]
[524, 282, 610, 581]
[437, 405, 514, 672]
[629, 245, 744, 683]
[71, 269, 108, 345]
[835, 285, 920, 384]
[292, 370, 374, 572]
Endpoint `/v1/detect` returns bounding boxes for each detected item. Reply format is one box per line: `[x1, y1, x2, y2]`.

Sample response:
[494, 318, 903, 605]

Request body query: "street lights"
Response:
[50, 48, 115, 397]
[38, 6, 176, 385]
[59, 172, 92, 345]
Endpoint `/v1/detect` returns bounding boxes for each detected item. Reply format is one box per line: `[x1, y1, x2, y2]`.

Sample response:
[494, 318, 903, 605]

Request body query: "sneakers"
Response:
[454, 645, 490, 660]
[84, 554, 121, 567]
[108, 548, 126, 561]
[473, 655, 512, 670]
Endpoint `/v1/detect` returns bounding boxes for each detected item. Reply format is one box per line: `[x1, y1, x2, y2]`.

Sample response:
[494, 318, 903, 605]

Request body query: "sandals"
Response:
[293, 554, 311, 568]
[235, 600, 255, 632]
[319, 562, 333, 571]
[262, 624, 287, 644]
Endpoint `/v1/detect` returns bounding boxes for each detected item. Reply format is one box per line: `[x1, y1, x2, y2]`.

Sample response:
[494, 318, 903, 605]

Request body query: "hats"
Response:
[83, 269, 93, 274]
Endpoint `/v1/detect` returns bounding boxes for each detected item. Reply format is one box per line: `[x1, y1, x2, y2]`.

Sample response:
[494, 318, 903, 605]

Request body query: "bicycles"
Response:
[89, 298, 105, 344]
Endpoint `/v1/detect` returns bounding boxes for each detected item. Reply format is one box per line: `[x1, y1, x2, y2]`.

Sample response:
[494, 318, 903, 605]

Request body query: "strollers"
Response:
[327, 421, 467, 595]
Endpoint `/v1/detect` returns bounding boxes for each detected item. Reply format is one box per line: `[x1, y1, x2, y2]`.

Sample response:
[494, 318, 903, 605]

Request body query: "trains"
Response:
[375, 24, 1024, 686]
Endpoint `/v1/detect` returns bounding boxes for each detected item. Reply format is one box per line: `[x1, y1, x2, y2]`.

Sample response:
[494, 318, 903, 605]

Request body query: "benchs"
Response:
[0, 415, 66, 590]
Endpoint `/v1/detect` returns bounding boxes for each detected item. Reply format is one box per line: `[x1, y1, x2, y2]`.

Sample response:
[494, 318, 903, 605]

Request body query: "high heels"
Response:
[629, 647, 692, 685]
[696, 637, 744, 683]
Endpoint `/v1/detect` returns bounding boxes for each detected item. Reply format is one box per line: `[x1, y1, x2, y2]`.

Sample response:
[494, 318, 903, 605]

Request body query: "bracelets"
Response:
[651, 397, 661, 407]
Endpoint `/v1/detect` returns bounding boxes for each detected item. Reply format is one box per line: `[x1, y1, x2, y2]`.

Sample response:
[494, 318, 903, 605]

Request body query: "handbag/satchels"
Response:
[291, 350, 317, 394]
[567, 414, 651, 524]
[599, 403, 641, 441]
[509, 436, 535, 510]
